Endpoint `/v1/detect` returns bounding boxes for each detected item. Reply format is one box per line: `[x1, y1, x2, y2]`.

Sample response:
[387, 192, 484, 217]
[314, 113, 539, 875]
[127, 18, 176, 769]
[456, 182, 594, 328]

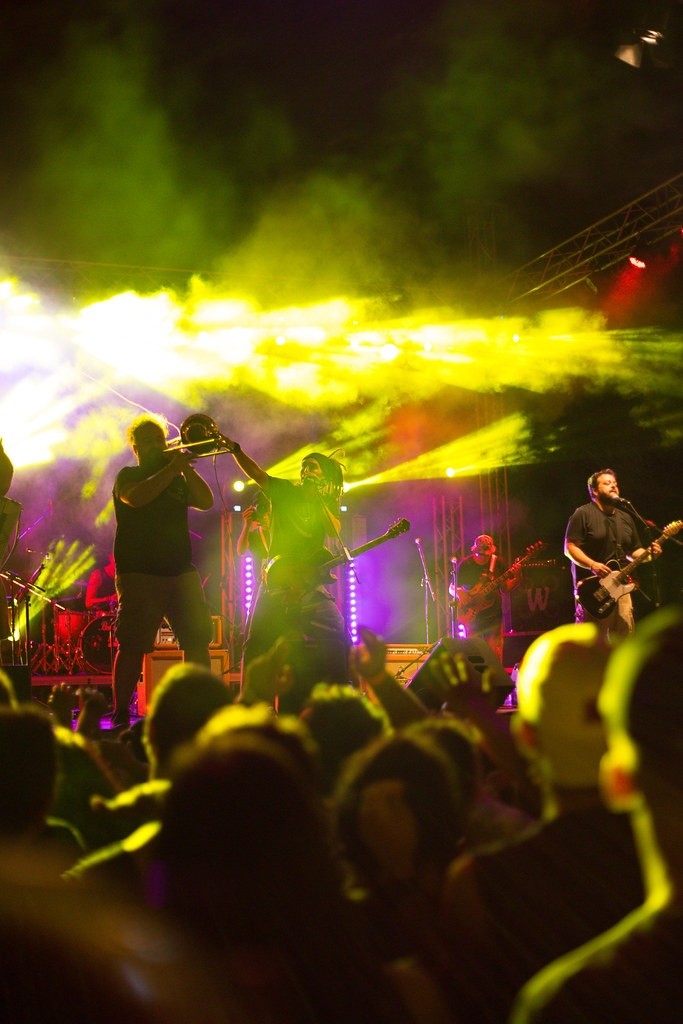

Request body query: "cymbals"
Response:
[72, 581, 94, 586]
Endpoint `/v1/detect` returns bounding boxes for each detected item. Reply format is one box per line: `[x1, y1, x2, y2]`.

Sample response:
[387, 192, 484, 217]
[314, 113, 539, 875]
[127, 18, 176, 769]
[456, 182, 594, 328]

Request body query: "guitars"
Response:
[576, 520, 683, 620]
[264, 518, 411, 610]
[457, 541, 545, 628]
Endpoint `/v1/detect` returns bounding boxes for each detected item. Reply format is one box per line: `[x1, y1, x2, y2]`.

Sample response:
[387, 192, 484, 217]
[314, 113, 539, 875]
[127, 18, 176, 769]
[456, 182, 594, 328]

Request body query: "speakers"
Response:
[404, 637, 517, 720]
[511, 567, 578, 633]
[360, 643, 436, 711]
[137, 649, 229, 720]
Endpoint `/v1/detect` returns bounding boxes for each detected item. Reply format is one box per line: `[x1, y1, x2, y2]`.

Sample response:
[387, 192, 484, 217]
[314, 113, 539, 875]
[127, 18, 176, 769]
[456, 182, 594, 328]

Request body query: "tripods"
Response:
[28, 594, 87, 677]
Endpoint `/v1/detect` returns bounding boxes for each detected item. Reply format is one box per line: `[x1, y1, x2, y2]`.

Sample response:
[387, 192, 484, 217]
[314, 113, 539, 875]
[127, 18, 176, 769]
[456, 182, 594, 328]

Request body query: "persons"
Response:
[218, 429, 349, 703]
[1, 607, 683, 1024]
[110, 420, 214, 724]
[448, 534, 522, 664]
[564, 469, 662, 642]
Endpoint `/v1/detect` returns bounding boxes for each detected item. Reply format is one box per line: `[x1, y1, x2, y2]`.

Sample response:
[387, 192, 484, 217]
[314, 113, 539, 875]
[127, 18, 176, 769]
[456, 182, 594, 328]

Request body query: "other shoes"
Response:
[110, 709, 129, 730]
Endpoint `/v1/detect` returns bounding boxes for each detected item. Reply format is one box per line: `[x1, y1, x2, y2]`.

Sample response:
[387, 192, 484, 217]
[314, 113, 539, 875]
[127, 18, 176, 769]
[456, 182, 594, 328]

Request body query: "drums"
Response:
[54, 611, 88, 655]
[78, 615, 119, 674]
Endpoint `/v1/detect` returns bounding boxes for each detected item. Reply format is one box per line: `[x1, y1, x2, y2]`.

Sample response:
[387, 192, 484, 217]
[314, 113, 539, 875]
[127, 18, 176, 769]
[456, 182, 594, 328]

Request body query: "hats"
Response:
[514, 624, 618, 787]
[302, 448, 347, 487]
[470, 535, 496, 555]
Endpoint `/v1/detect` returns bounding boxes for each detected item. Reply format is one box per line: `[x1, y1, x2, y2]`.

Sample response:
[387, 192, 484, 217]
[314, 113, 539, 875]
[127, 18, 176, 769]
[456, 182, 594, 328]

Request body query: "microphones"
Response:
[451, 557, 458, 563]
[613, 495, 627, 503]
[415, 538, 421, 545]
[304, 475, 325, 486]
[5, 570, 23, 581]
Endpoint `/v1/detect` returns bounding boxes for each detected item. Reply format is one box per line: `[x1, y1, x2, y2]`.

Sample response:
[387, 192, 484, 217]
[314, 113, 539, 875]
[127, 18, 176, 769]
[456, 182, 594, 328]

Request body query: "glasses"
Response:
[301, 462, 318, 470]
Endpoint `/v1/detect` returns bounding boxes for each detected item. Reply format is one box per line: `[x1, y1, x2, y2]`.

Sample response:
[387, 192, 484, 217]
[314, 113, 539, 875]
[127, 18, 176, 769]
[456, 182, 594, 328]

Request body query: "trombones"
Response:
[160, 414, 234, 459]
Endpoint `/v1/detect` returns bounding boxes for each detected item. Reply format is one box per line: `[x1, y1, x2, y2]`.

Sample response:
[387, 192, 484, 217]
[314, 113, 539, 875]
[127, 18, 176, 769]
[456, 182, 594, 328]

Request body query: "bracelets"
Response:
[231, 441, 240, 454]
[368, 669, 395, 692]
[590, 561, 595, 572]
[591, 561, 596, 571]
